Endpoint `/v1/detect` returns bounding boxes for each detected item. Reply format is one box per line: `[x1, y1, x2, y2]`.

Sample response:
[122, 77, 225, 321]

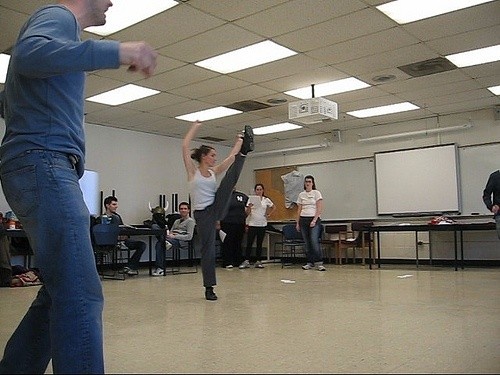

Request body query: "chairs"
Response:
[274, 222, 376, 264]
[93, 223, 127, 281]
[172, 224, 200, 267]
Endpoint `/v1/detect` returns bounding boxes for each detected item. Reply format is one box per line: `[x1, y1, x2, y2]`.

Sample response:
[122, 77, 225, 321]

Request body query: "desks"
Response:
[2, 190, 201, 276]
[261, 221, 297, 262]
[352, 219, 496, 271]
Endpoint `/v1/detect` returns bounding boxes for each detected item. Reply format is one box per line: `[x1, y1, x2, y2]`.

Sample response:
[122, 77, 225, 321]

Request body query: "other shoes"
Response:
[152, 267, 164, 276]
[223, 264, 234, 269]
[239, 260, 251, 269]
[205, 287, 217, 300]
[240, 125, 255, 155]
[315, 265, 325, 271]
[163, 241, 172, 251]
[302, 263, 314, 270]
[255, 261, 264, 268]
[122, 265, 138, 276]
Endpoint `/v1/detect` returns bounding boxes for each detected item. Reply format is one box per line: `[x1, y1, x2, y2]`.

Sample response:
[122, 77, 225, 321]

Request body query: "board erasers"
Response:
[472, 213, 479, 215]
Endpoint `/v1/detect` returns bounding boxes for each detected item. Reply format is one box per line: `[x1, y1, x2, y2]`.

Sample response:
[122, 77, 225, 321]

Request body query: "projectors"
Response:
[289, 97, 338, 124]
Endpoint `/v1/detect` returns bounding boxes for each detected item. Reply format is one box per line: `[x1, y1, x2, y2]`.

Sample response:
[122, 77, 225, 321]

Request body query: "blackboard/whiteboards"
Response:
[374, 142, 462, 217]
[298, 141, 500, 221]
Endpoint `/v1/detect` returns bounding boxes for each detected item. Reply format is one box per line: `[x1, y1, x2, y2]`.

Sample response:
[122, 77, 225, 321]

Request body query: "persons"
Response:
[0, 0, 158, 374]
[243, 184, 274, 268]
[183, 120, 255, 301]
[296, 175, 326, 271]
[151, 202, 195, 275]
[0, 212, 12, 269]
[216, 186, 249, 269]
[482, 169, 500, 239]
[95, 196, 147, 274]
